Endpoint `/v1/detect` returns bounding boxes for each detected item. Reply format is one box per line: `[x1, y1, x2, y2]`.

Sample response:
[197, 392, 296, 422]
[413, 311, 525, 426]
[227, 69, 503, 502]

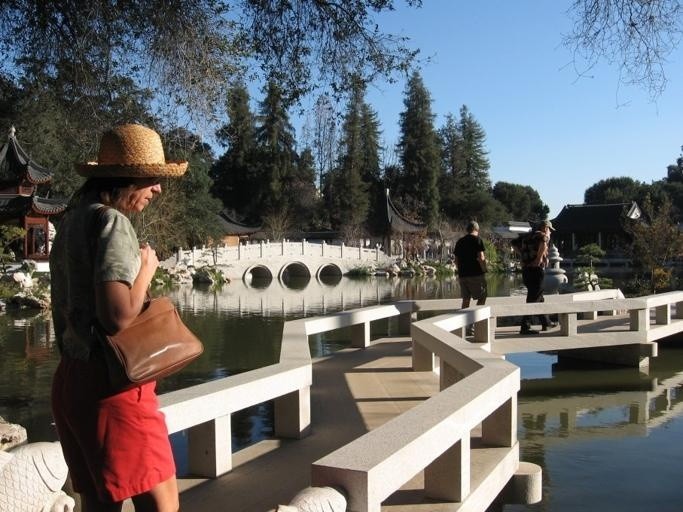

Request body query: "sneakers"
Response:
[542, 321, 559, 331]
[520, 326, 539, 337]
[465, 328, 474, 336]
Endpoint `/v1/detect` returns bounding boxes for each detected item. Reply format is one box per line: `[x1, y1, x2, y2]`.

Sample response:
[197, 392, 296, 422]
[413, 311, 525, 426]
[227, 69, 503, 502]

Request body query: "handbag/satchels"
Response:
[100, 296, 204, 383]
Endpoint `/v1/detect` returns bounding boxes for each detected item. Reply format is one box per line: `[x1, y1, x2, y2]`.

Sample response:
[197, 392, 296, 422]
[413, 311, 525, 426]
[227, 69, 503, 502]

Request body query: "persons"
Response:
[49, 124, 189, 512]
[454, 221, 487, 334]
[510, 219, 559, 336]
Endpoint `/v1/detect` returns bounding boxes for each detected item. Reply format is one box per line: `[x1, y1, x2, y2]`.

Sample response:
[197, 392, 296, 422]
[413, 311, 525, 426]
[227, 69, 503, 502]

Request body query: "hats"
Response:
[537, 220, 555, 230]
[74, 123, 188, 180]
